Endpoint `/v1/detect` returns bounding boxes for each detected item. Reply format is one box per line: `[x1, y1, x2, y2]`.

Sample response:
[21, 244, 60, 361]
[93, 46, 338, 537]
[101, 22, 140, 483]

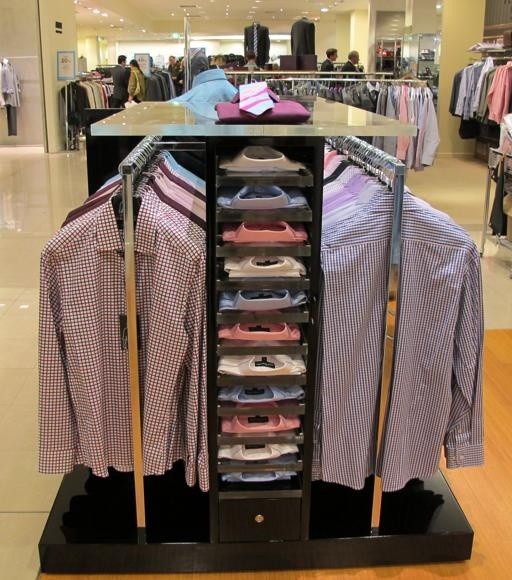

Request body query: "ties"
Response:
[371, 90, 378, 102]
[253, 26, 260, 61]
[238, 84, 274, 116]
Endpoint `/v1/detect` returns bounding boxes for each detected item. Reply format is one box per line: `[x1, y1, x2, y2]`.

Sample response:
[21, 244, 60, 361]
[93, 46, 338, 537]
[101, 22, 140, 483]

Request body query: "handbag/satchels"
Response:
[124, 101, 137, 107]
[129, 70, 142, 96]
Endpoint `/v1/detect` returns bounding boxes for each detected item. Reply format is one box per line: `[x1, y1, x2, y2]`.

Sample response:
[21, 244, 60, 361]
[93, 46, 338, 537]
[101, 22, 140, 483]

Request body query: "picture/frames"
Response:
[134, 54, 149, 75]
[58, 52, 74, 77]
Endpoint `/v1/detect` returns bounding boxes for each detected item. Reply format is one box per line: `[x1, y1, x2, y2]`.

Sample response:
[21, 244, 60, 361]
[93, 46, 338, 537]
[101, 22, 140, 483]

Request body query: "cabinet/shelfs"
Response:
[477, 147, 512, 281]
[218, 499, 303, 542]
[403, 34, 440, 79]
[375, 41, 400, 57]
[215, 144, 312, 488]
[377, 60, 400, 72]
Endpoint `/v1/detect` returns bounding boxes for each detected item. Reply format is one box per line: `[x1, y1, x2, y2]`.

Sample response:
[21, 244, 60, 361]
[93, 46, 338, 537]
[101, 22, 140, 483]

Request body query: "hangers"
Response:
[292, 78, 378, 96]
[324, 136, 408, 198]
[111, 136, 167, 230]
[378, 79, 428, 88]
[470, 55, 512, 62]
[262, 76, 430, 85]
[223, 70, 405, 76]
[467, 60, 512, 71]
[64, 77, 102, 87]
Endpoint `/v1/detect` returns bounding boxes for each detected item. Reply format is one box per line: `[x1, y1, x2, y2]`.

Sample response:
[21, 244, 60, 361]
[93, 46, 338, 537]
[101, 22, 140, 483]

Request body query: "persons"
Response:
[342, 51, 360, 81]
[128, 59, 145, 103]
[112, 55, 127, 109]
[243, 49, 259, 69]
[210, 54, 224, 69]
[167, 56, 180, 72]
[321, 49, 337, 84]
